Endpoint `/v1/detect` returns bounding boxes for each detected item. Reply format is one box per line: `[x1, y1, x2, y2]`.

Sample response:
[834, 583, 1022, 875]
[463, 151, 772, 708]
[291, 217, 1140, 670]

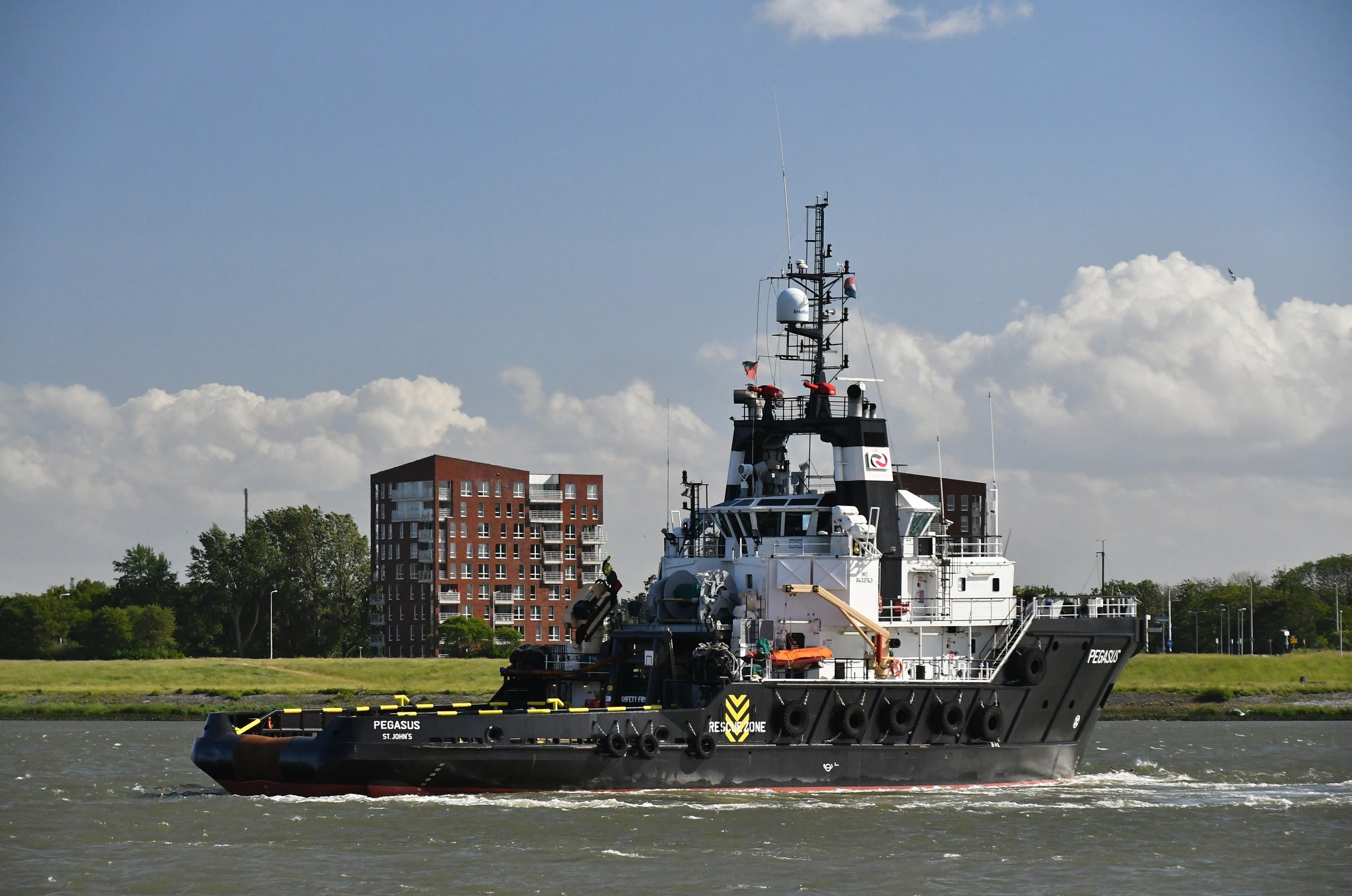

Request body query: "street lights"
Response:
[1146, 579, 1343, 658]
[59, 593, 70, 643]
[271, 590, 278, 659]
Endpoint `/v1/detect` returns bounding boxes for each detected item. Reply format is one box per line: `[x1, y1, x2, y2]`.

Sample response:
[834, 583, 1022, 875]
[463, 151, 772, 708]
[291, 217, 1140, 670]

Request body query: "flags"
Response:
[742, 360, 758, 380]
[844, 277, 858, 299]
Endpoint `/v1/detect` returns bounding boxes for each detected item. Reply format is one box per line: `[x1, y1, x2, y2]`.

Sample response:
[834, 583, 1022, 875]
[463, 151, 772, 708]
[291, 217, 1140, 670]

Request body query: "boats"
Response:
[193, 191, 1153, 797]
[739, 646, 833, 671]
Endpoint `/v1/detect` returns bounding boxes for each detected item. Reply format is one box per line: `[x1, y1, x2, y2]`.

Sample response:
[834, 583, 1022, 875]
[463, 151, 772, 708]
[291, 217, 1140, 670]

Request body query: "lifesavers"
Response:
[779, 700, 810, 737]
[886, 699, 917, 735]
[693, 731, 717, 759]
[635, 733, 659, 759]
[603, 732, 627, 758]
[891, 659, 903, 676]
[938, 699, 966, 735]
[879, 597, 882, 613]
[839, 702, 869, 737]
[978, 705, 1005, 739]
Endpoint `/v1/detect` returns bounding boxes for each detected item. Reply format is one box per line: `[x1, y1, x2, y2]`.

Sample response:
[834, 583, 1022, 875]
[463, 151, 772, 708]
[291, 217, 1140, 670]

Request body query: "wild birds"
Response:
[1110, 766, 1136, 776]
[1228, 705, 1256, 716]
[1228, 267, 1237, 282]
